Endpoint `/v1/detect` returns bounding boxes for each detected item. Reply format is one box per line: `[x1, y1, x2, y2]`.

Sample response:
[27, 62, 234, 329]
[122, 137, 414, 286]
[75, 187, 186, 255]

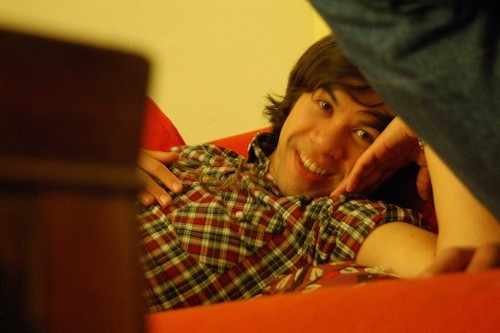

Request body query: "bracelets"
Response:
[418, 137, 427, 153]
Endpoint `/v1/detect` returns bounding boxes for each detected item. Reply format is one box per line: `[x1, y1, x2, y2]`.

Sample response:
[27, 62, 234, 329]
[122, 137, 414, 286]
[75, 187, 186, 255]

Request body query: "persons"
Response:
[136, 33, 500, 311]
